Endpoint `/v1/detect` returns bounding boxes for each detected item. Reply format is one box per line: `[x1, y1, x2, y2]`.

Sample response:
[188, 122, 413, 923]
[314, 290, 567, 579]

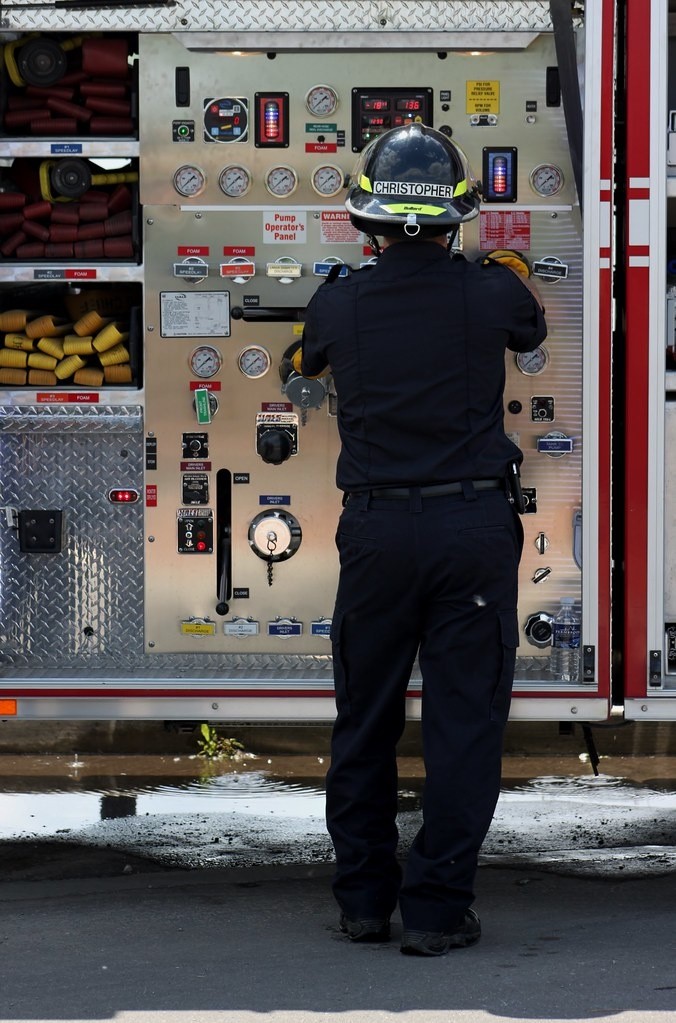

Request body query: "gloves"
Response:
[290, 347, 331, 379]
[482, 251, 531, 280]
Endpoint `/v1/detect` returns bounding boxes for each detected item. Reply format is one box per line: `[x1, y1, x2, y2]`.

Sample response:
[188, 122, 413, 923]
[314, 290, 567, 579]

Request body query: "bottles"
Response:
[550, 597, 581, 682]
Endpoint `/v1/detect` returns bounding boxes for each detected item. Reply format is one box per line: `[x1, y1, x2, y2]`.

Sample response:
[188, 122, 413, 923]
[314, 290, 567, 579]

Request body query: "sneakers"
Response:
[400, 916, 482, 956]
[339, 912, 391, 943]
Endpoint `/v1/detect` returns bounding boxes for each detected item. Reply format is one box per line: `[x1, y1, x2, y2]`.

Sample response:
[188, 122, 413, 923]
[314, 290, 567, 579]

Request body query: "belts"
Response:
[349, 480, 501, 500]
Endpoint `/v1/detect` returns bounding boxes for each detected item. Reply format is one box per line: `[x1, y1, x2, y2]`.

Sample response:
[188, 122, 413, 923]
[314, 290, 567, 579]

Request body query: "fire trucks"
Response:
[2, 2, 676, 791]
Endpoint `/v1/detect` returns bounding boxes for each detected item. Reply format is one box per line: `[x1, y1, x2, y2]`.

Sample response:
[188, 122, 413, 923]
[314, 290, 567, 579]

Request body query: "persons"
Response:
[293, 126, 547, 956]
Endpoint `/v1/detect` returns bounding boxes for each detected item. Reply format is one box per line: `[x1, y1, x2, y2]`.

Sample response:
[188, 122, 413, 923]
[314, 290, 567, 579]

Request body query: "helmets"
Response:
[345, 121, 480, 236]
